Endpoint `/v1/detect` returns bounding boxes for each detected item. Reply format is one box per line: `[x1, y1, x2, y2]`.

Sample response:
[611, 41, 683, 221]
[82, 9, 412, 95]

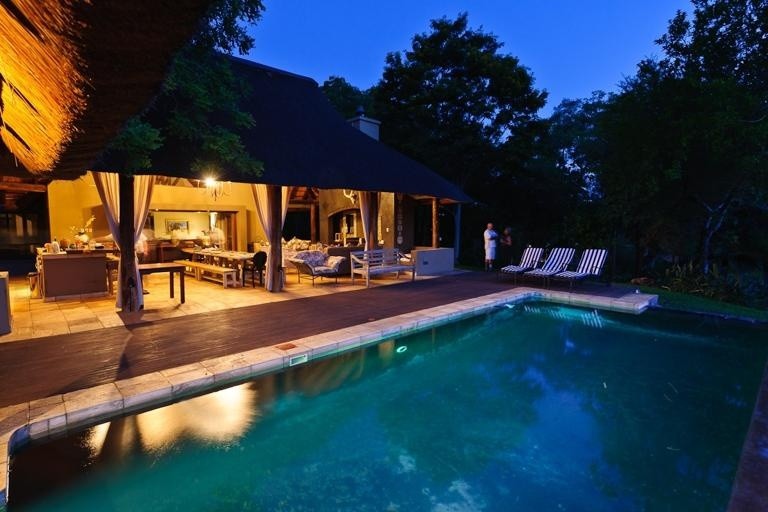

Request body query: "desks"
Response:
[138, 263, 186, 304]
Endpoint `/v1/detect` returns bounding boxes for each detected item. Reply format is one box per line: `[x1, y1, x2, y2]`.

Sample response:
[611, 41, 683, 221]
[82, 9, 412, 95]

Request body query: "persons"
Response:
[94, 232, 148, 296]
[483, 222, 498, 272]
[498, 226, 513, 265]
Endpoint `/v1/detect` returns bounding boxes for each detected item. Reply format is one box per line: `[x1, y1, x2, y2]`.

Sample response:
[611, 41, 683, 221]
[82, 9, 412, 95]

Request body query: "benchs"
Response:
[174, 248, 417, 289]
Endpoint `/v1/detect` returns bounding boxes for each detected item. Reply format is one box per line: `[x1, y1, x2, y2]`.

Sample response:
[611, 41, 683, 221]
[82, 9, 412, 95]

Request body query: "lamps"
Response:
[196, 179, 232, 202]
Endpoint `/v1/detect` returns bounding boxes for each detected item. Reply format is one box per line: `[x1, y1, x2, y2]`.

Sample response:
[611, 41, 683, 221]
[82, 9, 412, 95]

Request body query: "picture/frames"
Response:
[165, 219, 189, 235]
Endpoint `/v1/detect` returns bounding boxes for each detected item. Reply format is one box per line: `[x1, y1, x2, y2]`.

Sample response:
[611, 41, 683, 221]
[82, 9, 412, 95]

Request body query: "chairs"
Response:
[497, 247, 609, 293]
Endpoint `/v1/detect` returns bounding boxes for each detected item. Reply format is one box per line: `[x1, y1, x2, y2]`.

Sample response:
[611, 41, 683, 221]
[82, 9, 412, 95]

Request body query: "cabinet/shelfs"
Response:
[143, 238, 202, 264]
